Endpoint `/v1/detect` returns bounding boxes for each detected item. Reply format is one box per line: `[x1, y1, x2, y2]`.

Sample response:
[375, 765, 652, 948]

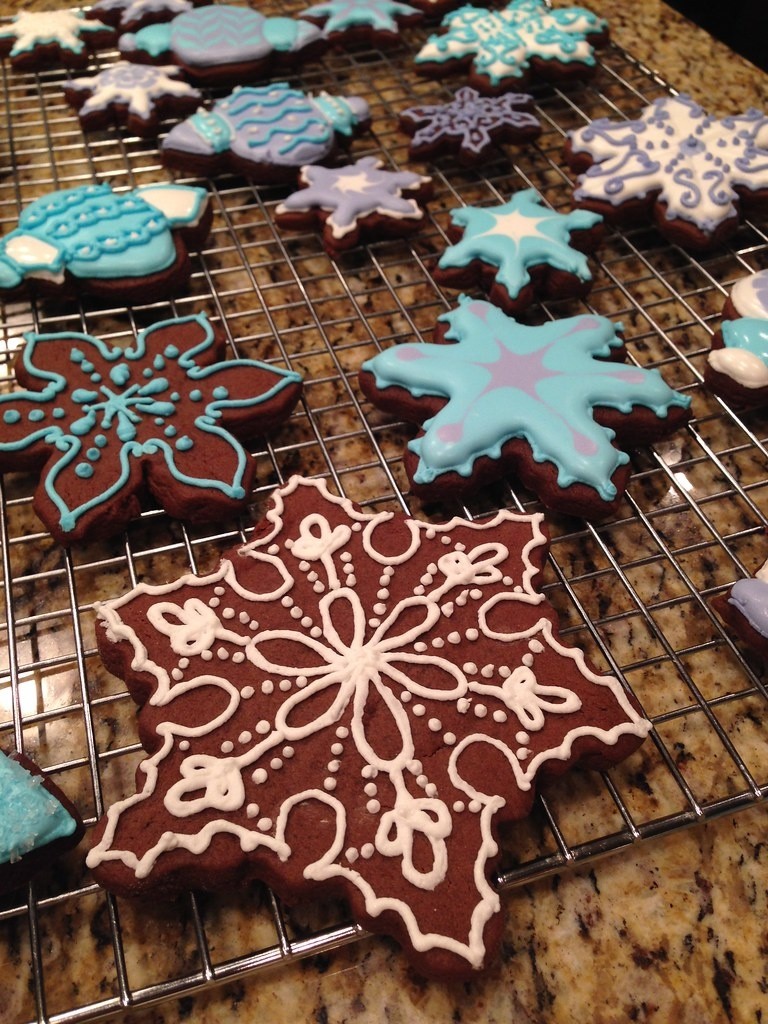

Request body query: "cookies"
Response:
[0, 0, 768, 986]
